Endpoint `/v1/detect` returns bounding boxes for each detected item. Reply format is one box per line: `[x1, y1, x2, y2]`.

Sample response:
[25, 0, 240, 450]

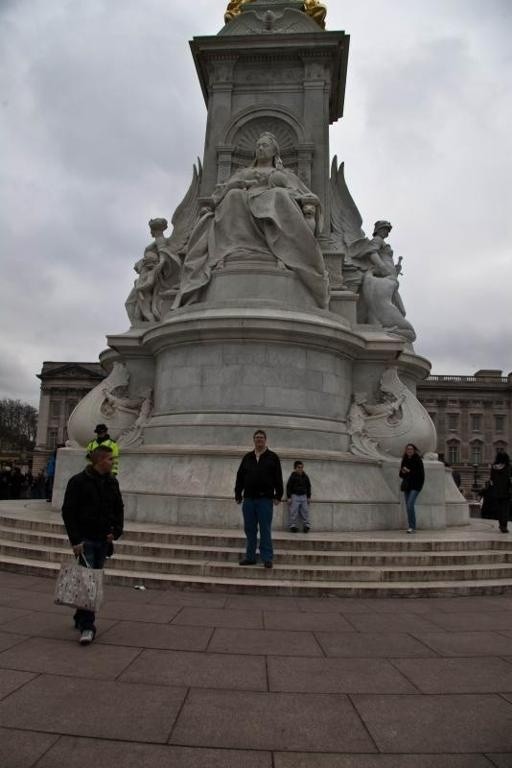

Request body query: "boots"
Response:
[499, 519, 509, 533]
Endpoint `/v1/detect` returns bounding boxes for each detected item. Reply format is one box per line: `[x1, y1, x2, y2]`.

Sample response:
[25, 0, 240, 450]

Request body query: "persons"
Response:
[478, 480, 496, 519]
[205, 128, 322, 218]
[60, 445, 125, 646]
[83, 424, 120, 477]
[126, 212, 178, 326]
[1, 443, 66, 503]
[368, 218, 408, 317]
[399, 443, 425, 534]
[234, 429, 285, 568]
[286, 460, 312, 533]
[490, 450, 512, 533]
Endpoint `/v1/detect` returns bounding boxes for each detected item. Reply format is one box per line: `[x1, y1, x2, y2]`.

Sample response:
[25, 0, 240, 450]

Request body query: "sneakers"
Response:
[303, 526, 310, 533]
[46, 499, 52, 502]
[291, 527, 299, 533]
[406, 528, 416, 534]
[263, 560, 273, 569]
[74, 624, 94, 644]
[239, 559, 256, 565]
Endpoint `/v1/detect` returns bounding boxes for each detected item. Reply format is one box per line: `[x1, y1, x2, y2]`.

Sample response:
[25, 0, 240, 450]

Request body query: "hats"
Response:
[94, 424, 108, 433]
[444, 461, 453, 467]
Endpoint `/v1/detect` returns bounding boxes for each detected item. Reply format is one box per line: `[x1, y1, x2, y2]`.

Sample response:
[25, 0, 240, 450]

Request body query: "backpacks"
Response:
[479, 482, 500, 499]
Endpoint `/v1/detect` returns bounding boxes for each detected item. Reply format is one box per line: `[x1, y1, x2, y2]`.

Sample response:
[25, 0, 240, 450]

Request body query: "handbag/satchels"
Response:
[54, 551, 104, 613]
